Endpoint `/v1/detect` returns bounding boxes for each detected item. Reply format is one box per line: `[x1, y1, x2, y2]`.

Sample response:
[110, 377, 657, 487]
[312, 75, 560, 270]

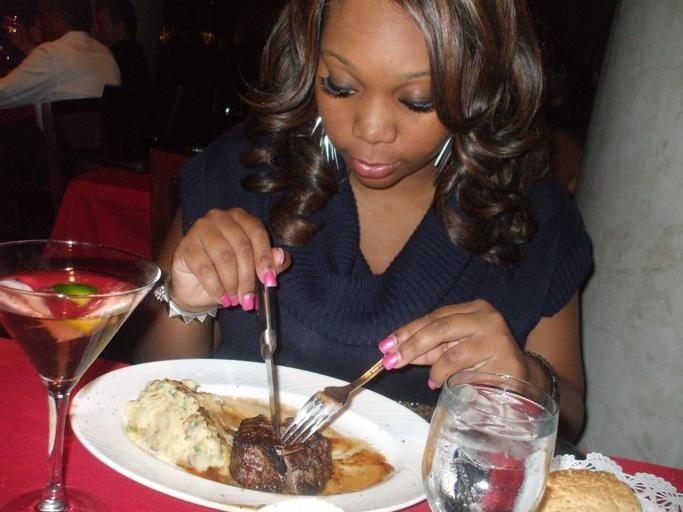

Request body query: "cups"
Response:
[420, 370, 558, 512]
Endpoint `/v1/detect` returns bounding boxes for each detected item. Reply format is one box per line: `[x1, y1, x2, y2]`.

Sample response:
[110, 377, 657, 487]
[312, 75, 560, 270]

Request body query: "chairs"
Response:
[0, 80, 184, 250]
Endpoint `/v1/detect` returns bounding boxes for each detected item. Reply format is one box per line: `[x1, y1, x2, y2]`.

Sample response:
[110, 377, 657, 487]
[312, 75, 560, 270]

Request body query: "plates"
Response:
[70, 358, 439, 512]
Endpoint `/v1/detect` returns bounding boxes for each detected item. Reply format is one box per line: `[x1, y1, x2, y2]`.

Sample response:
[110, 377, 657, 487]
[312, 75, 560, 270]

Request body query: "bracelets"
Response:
[152, 270, 219, 325]
[523, 350, 561, 417]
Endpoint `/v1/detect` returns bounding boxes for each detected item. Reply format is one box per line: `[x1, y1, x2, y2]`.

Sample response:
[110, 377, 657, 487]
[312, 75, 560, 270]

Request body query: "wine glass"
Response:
[1, 239, 163, 512]
[0, 10, 24, 62]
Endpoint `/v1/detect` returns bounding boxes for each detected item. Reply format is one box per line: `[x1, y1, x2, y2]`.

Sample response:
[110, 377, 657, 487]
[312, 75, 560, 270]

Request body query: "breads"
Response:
[535, 468, 642, 512]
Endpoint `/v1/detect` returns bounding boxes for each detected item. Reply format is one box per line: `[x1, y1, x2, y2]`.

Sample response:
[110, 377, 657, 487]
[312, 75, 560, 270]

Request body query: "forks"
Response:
[281, 349, 386, 446]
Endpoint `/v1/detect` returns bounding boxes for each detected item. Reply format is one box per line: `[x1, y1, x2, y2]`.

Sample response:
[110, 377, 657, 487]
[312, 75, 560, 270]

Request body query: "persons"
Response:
[115, 1, 596, 445]
[1, 0, 122, 180]
[29, 0, 155, 88]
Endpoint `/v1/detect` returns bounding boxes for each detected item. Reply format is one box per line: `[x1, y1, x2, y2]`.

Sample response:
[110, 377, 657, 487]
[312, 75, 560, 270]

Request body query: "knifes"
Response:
[256, 269, 284, 440]
